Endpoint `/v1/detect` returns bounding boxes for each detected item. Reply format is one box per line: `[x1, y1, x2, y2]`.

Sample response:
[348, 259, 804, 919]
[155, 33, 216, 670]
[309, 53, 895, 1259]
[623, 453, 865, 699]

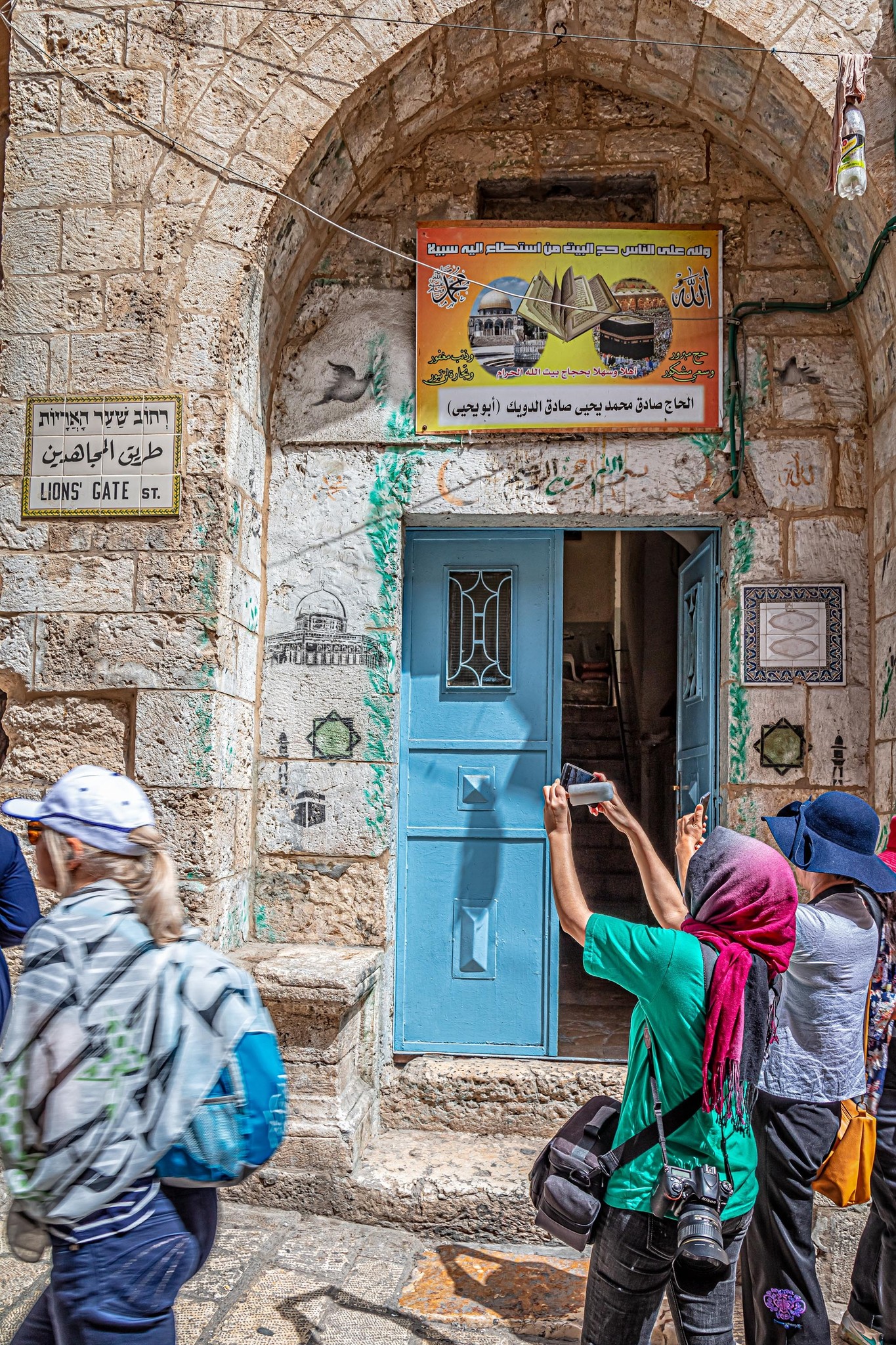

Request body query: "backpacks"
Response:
[140, 930, 286, 1188]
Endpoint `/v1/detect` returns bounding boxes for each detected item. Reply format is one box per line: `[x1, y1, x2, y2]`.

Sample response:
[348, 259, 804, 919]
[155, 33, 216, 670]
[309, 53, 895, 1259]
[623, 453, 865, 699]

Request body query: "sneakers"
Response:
[838, 1310, 883, 1345]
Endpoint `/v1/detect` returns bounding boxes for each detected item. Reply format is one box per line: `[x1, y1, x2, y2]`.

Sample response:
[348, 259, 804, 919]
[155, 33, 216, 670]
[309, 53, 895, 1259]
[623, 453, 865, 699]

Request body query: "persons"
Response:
[542, 772, 798, 1345]
[0, 690, 219, 1345]
[675, 791, 896, 1345]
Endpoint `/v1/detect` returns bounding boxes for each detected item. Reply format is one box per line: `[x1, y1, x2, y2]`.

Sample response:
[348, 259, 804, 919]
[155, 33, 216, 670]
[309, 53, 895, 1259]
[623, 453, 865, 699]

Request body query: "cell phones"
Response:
[559, 763, 600, 808]
[696, 792, 710, 844]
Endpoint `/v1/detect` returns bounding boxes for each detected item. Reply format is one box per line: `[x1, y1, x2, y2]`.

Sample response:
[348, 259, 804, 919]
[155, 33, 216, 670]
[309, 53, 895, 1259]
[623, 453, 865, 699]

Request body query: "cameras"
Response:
[651, 1164, 736, 1298]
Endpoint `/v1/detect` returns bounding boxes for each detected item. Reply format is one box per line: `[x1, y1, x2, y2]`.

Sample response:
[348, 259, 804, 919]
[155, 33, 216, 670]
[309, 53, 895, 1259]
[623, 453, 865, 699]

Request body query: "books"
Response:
[515, 266, 622, 342]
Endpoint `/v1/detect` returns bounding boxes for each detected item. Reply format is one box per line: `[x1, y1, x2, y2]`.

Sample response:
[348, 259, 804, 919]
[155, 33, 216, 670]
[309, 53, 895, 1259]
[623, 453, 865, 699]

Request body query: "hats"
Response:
[761, 791, 896, 893]
[2, 765, 155, 856]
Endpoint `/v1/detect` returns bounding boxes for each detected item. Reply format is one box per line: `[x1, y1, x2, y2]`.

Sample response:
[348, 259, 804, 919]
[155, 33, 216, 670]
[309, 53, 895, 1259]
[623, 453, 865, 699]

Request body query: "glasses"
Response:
[27, 820, 46, 846]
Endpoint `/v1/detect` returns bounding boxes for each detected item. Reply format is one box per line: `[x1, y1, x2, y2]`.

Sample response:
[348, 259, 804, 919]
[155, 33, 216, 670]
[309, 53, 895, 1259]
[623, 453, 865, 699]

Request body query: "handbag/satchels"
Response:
[813, 1099, 876, 1207]
[530, 1095, 622, 1253]
